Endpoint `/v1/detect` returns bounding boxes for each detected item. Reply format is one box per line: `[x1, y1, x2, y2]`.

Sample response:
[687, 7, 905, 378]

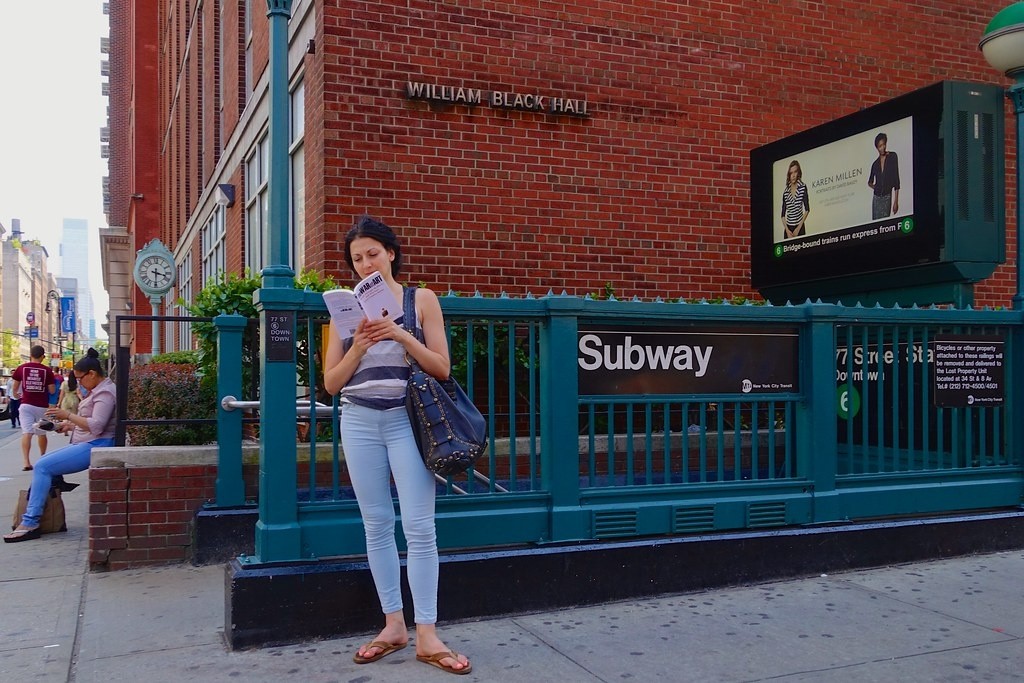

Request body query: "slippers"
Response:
[416, 649, 472, 674]
[3, 526, 41, 543]
[353, 640, 408, 664]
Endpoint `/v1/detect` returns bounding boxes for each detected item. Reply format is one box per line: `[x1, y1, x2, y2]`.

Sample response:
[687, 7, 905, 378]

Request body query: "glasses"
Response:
[43, 355, 45, 358]
[74, 368, 94, 383]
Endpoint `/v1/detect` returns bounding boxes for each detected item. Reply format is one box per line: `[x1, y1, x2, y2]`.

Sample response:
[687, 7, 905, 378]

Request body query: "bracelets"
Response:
[67, 412, 71, 420]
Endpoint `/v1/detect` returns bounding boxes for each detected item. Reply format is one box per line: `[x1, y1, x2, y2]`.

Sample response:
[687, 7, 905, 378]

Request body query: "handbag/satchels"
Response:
[13, 483, 68, 534]
[403, 287, 488, 477]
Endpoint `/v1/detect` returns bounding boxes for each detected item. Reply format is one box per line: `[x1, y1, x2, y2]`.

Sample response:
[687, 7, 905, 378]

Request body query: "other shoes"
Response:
[65, 434, 68, 436]
[22, 465, 33, 471]
[12, 425, 16, 428]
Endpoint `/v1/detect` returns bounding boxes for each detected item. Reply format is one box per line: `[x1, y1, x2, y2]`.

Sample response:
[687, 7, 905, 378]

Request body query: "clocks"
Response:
[138, 256, 172, 288]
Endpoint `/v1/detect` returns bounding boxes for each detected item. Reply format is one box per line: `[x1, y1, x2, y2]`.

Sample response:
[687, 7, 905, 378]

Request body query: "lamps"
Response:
[214, 184, 236, 208]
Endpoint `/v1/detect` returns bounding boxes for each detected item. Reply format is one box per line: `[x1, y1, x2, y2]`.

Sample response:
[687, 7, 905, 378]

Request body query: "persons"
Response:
[868, 133, 900, 220]
[2, 345, 116, 542]
[323, 217, 473, 676]
[779, 160, 811, 240]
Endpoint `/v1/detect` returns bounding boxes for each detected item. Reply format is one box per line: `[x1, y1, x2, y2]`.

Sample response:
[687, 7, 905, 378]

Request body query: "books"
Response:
[322, 271, 405, 340]
